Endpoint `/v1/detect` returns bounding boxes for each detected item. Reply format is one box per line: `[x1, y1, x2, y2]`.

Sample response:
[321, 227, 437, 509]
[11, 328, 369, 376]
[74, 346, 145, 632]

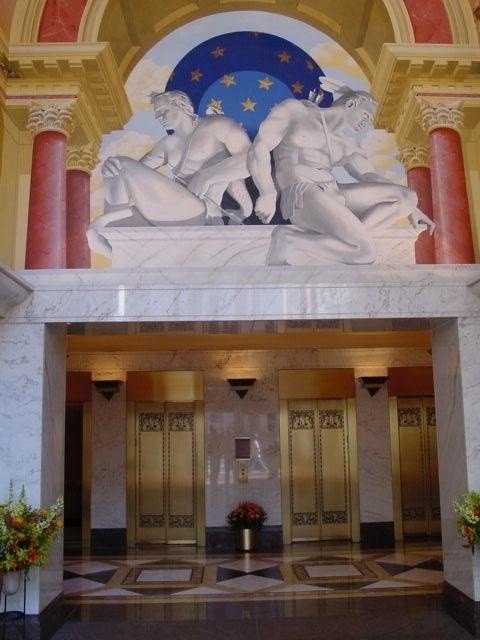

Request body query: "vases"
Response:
[239, 529, 256, 551]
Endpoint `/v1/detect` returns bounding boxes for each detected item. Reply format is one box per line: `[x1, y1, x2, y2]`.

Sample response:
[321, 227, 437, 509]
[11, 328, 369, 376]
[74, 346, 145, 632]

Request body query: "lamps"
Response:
[91, 380, 122, 400]
[356, 376, 389, 396]
[227, 378, 256, 399]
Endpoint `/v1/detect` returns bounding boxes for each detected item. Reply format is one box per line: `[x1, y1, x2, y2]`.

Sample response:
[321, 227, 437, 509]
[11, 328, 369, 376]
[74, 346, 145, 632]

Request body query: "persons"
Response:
[245, 91, 436, 266]
[87, 89, 253, 258]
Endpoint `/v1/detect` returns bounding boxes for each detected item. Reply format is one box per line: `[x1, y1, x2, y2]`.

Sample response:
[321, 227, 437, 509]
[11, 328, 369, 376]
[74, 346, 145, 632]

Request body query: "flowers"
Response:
[0, 478, 64, 583]
[446, 488, 480, 555]
[224, 501, 269, 529]
